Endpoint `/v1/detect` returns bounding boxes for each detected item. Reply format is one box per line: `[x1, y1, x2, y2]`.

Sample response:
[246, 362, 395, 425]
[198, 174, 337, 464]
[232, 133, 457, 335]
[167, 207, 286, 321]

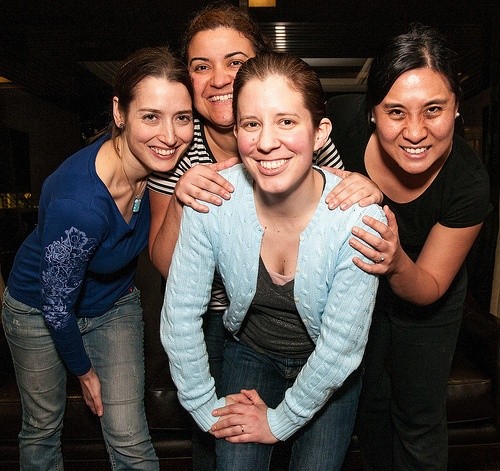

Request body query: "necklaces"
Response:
[117, 137, 144, 212]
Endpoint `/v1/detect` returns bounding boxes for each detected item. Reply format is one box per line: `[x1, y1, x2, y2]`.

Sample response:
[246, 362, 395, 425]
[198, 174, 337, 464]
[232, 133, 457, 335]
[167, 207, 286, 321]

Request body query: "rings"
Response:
[375, 252, 385, 263]
[241, 425, 245, 435]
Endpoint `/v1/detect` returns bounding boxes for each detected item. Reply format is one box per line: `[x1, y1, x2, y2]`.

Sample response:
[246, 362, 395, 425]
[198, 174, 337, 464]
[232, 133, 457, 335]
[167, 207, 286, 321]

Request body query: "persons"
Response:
[146, 9, 384, 471]
[1, 46, 194, 471]
[324, 28, 494, 471]
[160, 52, 388, 471]
